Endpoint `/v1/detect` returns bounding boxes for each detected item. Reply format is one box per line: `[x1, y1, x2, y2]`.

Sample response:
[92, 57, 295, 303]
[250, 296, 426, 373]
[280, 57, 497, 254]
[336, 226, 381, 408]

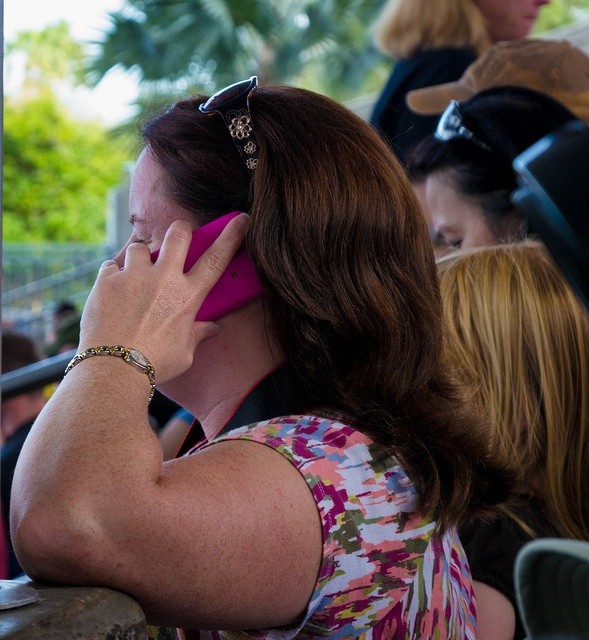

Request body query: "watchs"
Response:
[64, 345, 155, 406]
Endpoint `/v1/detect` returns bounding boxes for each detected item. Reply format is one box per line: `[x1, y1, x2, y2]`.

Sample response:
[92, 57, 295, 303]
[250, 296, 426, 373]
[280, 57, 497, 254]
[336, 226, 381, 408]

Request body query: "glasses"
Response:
[198, 77, 259, 169]
[433, 100, 511, 171]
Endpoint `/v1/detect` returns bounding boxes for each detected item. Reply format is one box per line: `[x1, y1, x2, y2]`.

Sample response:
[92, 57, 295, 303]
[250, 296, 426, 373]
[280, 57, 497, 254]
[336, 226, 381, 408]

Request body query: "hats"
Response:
[407, 41, 589, 128]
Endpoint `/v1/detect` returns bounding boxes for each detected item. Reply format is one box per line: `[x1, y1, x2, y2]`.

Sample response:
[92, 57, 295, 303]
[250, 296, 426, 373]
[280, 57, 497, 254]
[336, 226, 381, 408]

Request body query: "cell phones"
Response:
[119, 210, 266, 321]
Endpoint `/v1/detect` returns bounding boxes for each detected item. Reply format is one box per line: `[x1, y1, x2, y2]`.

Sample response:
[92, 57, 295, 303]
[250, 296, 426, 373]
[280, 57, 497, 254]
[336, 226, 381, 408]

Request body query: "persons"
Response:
[10, 84, 478, 639]
[437, 243, 588, 639]
[1, 332, 47, 580]
[428, 89, 585, 253]
[42, 301, 82, 354]
[367, 1, 589, 161]
